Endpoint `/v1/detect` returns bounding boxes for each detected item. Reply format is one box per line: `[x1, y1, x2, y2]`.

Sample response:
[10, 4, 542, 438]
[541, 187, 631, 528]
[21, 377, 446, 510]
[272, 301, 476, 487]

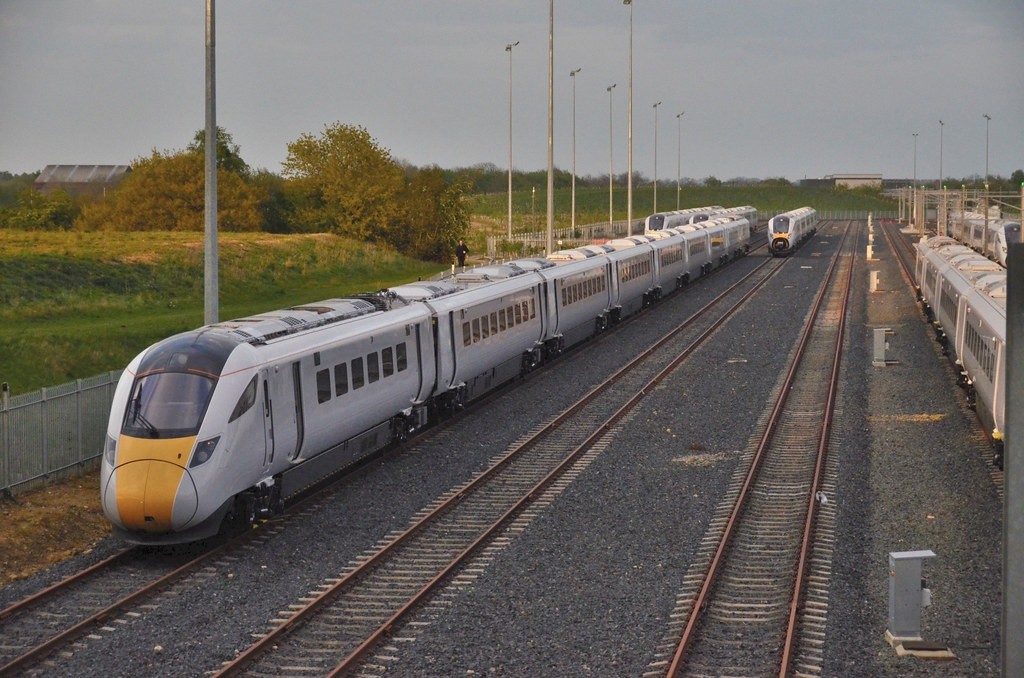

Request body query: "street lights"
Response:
[677, 110, 685, 211]
[912, 132, 918, 188]
[982, 113, 991, 257]
[569, 66, 582, 231]
[652, 100, 662, 214]
[505, 41, 519, 236]
[607, 82, 617, 225]
[938, 119, 945, 188]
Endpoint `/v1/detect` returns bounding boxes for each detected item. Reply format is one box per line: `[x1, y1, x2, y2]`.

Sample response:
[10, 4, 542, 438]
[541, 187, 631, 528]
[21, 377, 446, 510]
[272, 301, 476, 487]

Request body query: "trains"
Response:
[949, 210, 1023, 268]
[914, 235, 1008, 457]
[767, 206, 818, 257]
[100, 214, 751, 546]
[644, 206, 758, 235]
